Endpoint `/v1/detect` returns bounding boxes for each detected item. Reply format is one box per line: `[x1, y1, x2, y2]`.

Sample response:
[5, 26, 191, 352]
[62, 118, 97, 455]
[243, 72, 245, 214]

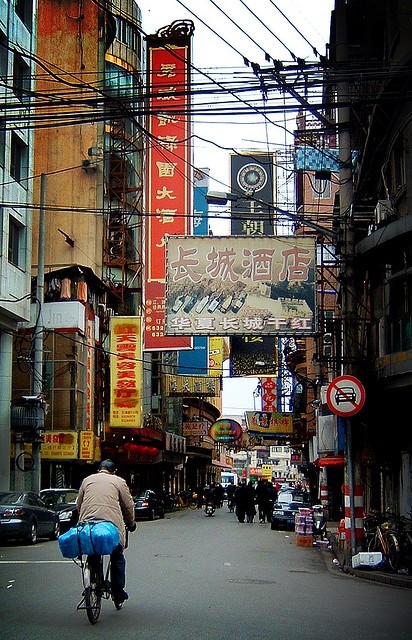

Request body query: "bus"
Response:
[221, 472, 241, 500]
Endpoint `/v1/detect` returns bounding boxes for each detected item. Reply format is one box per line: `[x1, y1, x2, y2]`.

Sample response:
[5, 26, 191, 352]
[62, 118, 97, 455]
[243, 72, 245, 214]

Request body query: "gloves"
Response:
[128, 523, 136, 532]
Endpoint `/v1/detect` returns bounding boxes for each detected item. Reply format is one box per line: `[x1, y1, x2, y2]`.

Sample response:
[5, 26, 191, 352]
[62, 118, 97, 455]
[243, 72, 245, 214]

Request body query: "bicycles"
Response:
[75, 517, 137, 625]
[164, 488, 206, 511]
[363, 507, 411, 573]
[226, 493, 234, 513]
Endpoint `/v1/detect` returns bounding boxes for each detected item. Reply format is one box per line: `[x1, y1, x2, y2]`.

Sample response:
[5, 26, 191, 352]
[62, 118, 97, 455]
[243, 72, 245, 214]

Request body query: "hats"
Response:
[98, 458, 114, 472]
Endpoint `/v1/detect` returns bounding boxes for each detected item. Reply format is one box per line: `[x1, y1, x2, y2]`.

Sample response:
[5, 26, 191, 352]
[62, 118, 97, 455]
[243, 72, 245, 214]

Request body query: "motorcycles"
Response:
[204, 498, 215, 516]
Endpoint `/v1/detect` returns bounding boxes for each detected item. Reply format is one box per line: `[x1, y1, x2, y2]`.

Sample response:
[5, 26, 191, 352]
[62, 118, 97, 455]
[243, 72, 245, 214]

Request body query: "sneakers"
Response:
[113, 590, 128, 603]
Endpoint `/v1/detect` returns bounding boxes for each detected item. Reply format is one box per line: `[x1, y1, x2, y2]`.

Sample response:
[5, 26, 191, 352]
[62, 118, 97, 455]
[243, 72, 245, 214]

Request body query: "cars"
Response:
[131, 489, 165, 520]
[38, 488, 76, 499]
[0, 489, 61, 545]
[38, 487, 83, 539]
[271, 488, 313, 531]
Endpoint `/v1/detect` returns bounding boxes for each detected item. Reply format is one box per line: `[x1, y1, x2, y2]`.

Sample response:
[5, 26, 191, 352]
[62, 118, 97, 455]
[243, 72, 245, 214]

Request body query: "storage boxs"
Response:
[296, 534, 314, 548]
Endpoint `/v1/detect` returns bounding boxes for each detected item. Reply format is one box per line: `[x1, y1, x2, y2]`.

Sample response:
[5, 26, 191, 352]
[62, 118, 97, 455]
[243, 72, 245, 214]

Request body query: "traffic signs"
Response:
[326, 376, 366, 419]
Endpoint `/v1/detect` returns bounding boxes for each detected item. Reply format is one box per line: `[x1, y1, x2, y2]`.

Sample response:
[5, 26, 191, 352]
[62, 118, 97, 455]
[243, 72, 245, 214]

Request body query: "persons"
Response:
[76, 458, 137, 600]
[196, 480, 278, 523]
